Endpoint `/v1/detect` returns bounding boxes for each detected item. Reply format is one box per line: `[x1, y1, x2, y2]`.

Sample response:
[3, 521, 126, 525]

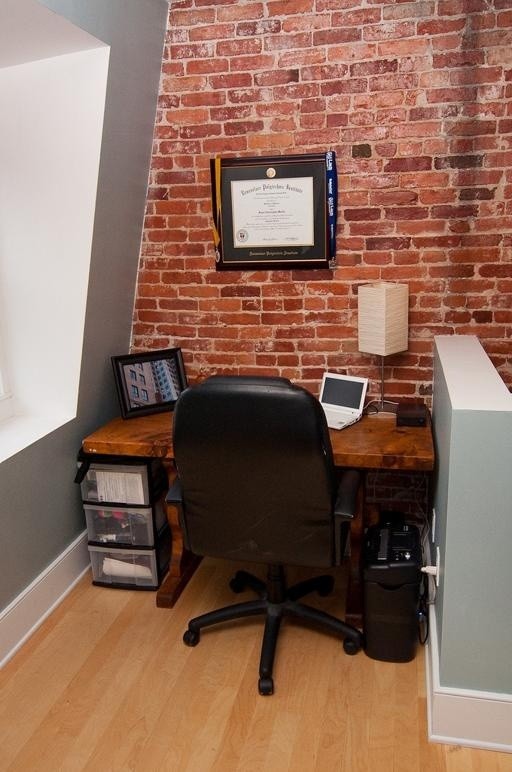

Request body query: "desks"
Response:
[81, 406, 435, 609]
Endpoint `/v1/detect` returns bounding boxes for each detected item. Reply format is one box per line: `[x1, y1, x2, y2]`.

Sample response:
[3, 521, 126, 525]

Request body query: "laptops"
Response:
[318, 372, 368, 431]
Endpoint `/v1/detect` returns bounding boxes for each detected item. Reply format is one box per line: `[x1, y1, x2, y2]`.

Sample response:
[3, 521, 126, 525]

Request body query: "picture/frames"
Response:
[210, 152, 335, 272]
[111, 346, 188, 420]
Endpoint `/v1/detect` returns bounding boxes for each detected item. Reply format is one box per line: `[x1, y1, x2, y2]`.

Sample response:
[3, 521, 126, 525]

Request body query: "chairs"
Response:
[172, 375, 361, 692]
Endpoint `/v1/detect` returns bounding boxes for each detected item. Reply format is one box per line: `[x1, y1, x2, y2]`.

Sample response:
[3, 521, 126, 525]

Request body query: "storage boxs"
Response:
[77, 455, 168, 507]
[81, 494, 166, 547]
[86, 538, 168, 591]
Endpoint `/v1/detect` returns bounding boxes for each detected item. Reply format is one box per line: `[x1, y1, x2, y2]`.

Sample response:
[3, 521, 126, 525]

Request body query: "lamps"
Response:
[357, 282, 409, 412]
